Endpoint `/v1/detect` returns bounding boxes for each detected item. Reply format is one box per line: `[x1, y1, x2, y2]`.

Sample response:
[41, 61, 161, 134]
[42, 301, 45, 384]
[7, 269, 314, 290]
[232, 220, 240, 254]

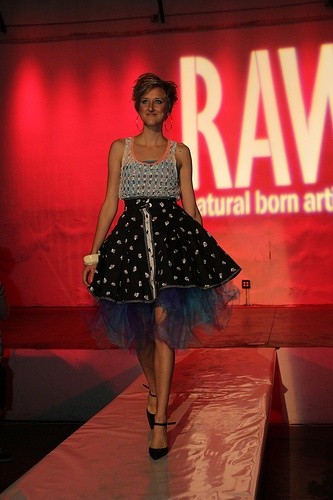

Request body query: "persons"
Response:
[83, 72, 242, 461]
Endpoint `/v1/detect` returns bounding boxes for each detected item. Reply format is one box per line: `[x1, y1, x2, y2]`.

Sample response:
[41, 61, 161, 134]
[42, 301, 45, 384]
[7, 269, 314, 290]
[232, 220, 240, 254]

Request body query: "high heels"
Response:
[141, 384, 158, 427]
[148, 420, 176, 460]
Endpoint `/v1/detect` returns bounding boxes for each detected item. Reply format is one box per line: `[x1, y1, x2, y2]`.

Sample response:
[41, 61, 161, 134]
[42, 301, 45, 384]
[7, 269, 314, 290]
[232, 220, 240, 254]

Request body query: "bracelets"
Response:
[81, 253, 100, 265]
